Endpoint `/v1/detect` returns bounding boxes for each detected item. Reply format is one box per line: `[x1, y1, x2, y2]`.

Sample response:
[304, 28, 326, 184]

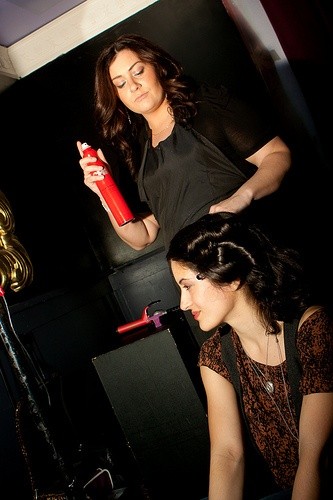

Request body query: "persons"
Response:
[166, 211, 333, 500]
[76, 35, 292, 251]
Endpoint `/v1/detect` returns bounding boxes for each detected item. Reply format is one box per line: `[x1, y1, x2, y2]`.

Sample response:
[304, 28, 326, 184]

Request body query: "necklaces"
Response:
[246, 332, 273, 393]
[150, 111, 174, 139]
[243, 330, 299, 442]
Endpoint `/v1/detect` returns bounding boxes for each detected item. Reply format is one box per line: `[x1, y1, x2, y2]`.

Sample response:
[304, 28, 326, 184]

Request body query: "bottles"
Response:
[79, 142, 135, 228]
